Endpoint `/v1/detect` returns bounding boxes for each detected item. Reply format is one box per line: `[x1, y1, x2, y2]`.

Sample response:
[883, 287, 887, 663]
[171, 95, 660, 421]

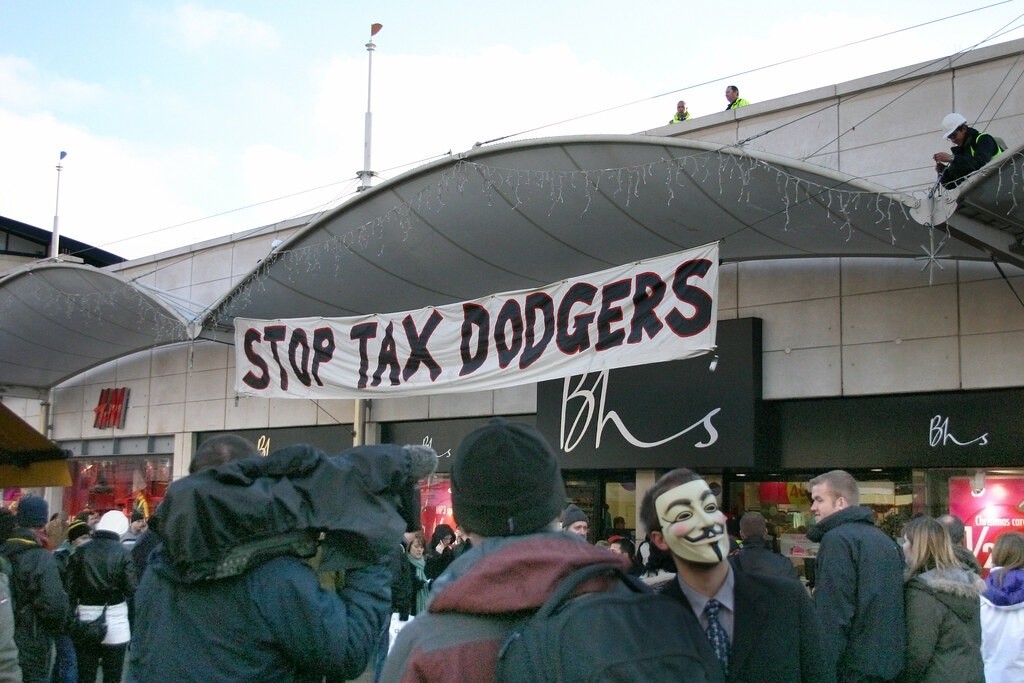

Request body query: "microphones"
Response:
[402, 445, 438, 480]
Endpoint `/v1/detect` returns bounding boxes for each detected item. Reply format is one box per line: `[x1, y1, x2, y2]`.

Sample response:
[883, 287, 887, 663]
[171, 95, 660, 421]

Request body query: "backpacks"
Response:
[493, 562, 708, 683]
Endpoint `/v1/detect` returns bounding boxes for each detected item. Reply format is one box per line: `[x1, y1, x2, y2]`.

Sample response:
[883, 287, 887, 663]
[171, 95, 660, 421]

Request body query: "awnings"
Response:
[0, 401, 73, 488]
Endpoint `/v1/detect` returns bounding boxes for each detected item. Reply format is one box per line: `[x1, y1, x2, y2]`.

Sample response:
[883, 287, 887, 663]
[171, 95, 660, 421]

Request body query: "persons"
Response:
[725, 85, 749, 110]
[669, 100, 693, 125]
[933, 113, 1003, 190]
[0, 416, 1024, 683]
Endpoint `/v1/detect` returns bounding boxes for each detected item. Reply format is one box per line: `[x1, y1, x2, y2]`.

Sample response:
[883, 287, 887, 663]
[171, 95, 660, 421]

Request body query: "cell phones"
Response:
[439, 540, 442, 545]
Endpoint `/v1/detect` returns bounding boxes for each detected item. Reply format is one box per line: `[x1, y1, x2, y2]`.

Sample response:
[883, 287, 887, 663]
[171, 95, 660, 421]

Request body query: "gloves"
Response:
[66, 618, 89, 638]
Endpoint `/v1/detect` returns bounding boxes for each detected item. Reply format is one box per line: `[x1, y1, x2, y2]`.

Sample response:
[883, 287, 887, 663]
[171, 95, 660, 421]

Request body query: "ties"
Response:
[704, 598, 731, 680]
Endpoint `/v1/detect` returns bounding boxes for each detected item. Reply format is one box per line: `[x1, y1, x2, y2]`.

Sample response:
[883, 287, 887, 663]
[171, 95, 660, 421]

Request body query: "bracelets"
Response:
[951, 155, 954, 161]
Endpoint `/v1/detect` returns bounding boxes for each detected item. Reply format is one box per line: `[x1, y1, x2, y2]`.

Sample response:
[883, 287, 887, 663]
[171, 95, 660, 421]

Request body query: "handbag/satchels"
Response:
[66, 610, 107, 646]
[14, 626, 57, 683]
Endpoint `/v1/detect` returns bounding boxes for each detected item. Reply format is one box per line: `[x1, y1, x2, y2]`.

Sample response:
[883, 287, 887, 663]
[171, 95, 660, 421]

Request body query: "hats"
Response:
[96, 510, 129, 538]
[739, 512, 767, 538]
[562, 503, 590, 530]
[18, 495, 49, 528]
[450, 416, 565, 536]
[131, 510, 144, 523]
[68, 519, 92, 541]
